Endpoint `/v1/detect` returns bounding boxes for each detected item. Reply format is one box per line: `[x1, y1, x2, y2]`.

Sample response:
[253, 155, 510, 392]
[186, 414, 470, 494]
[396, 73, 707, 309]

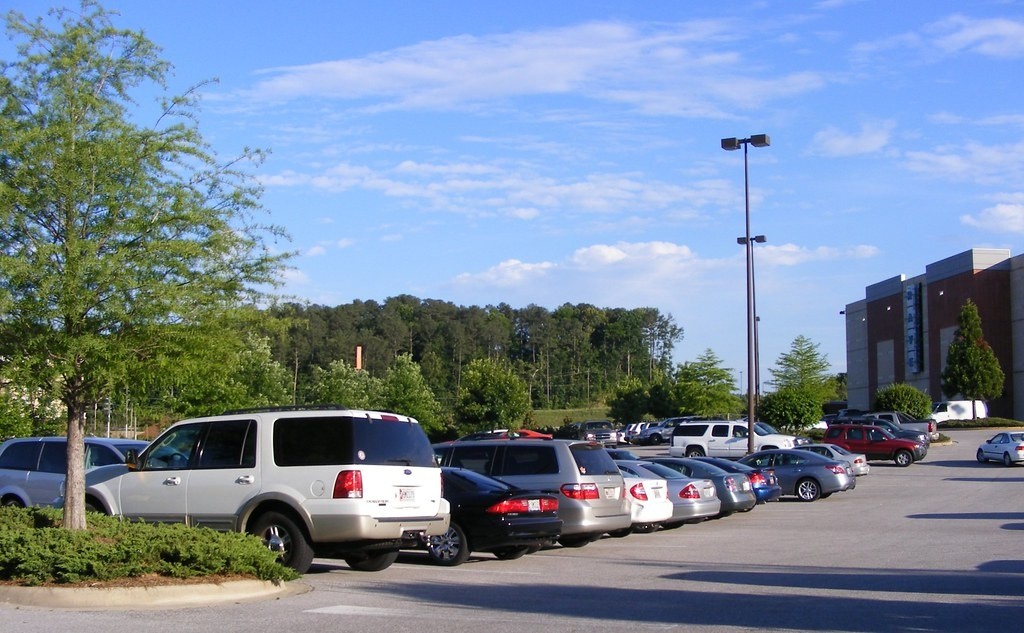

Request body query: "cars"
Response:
[781, 409, 861, 429]
[637, 458, 756, 520]
[736, 449, 856, 502]
[578, 416, 703, 449]
[976, 432, 1024, 468]
[428, 467, 564, 567]
[607, 469, 673, 538]
[690, 458, 782, 512]
[613, 460, 722, 529]
[456, 429, 553, 440]
[761, 444, 869, 476]
[606, 448, 639, 460]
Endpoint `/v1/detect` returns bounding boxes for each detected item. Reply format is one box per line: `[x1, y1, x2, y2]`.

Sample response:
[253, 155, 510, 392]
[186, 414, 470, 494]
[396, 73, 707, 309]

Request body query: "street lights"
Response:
[720, 134, 771, 455]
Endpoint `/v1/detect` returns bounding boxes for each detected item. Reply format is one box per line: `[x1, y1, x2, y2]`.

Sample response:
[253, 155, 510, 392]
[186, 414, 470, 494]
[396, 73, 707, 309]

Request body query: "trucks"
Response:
[925, 399, 986, 423]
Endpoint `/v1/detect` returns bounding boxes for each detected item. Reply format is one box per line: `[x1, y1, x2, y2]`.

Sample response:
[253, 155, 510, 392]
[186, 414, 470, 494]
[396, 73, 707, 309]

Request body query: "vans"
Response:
[0, 436, 188, 510]
[432, 436, 630, 548]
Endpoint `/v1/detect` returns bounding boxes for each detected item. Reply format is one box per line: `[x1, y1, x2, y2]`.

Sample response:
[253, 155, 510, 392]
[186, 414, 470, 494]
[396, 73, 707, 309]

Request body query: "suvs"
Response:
[84, 403, 450, 576]
[823, 417, 929, 467]
[669, 420, 813, 461]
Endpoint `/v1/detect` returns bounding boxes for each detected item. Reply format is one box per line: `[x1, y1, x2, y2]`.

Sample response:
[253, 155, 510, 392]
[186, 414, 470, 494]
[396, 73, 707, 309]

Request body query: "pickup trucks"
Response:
[862, 413, 939, 441]
